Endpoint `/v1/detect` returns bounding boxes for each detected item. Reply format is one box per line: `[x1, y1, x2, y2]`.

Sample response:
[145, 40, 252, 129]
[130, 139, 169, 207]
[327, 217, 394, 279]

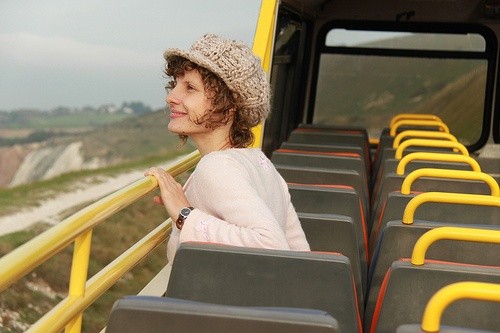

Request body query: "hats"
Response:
[163, 31, 272, 128]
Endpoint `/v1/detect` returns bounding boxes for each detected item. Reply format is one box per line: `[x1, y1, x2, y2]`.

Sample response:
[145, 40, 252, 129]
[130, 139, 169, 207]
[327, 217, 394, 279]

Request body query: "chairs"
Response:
[102, 111, 500, 333]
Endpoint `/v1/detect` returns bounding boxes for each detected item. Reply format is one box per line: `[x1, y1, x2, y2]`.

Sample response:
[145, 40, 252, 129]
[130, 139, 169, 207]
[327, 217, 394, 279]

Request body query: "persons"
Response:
[144, 33, 311, 267]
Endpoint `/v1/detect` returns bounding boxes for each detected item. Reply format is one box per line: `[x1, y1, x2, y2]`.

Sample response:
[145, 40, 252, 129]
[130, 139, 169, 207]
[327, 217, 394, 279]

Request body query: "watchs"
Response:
[176, 206, 194, 230]
